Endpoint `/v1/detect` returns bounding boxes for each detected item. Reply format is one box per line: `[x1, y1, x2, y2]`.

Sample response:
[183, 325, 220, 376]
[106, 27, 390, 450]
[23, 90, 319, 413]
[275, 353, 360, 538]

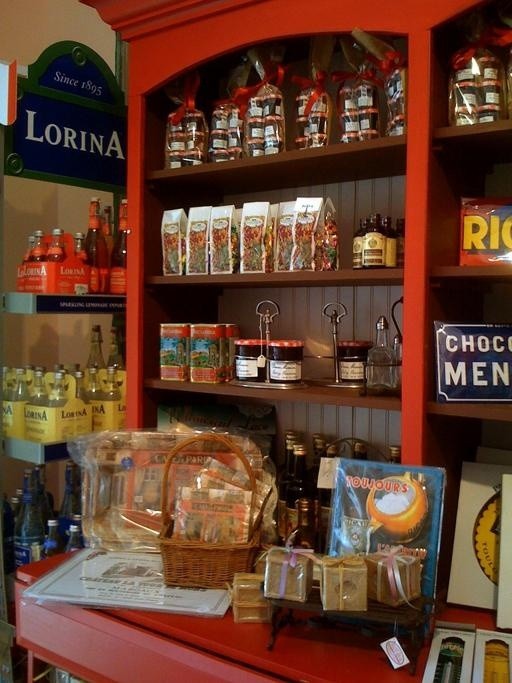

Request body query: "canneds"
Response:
[269, 339, 304, 383]
[191, 324, 229, 383]
[336, 340, 373, 383]
[160, 323, 190, 382]
[227, 325, 241, 379]
[234, 339, 267, 382]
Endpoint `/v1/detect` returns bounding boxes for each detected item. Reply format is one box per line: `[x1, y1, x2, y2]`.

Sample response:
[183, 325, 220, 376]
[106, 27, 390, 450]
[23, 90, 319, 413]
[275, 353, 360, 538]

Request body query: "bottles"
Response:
[273, 428, 401, 554]
[431, 635, 466, 682]
[483, 638, 510, 682]
[22, 196, 127, 295]
[3, 462, 84, 569]
[0, 323, 125, 410]
[366, 313, 402, 394]
[352, 214, 404, 269]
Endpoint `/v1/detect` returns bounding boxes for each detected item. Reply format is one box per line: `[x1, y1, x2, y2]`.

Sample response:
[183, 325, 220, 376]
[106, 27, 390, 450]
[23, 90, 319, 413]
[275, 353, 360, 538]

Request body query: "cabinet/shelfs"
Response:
[7, 0, 512, 683]
[0, 40, 125, 467]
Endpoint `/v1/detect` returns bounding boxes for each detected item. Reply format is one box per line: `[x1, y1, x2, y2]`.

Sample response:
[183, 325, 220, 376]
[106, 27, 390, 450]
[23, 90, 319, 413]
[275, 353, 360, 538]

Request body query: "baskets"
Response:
[156, 432, 260, 589]
